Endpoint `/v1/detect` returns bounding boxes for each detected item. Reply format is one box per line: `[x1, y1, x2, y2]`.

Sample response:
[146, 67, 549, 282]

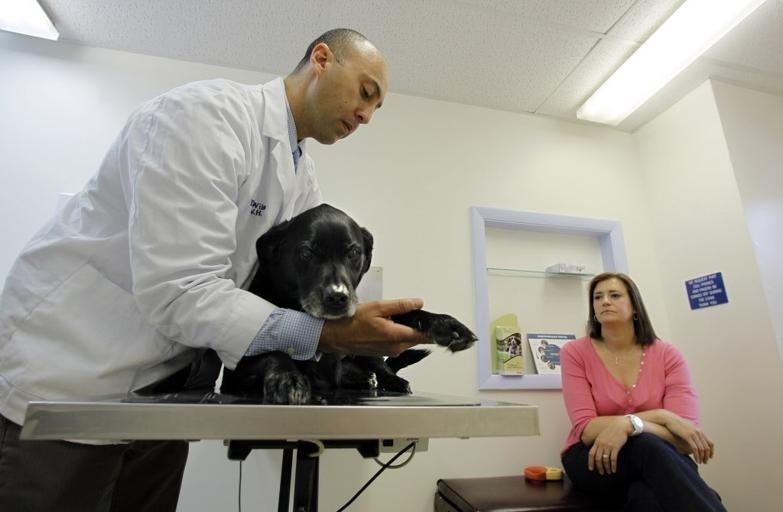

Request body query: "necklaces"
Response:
[598, 339, 638, 367]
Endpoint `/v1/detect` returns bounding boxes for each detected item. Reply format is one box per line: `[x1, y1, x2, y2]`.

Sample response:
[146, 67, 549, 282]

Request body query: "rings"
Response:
[602, 453, 609, 460]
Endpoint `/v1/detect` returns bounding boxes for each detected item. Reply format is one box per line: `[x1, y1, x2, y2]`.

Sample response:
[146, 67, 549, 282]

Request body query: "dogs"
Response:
[218, 202, 480, 405]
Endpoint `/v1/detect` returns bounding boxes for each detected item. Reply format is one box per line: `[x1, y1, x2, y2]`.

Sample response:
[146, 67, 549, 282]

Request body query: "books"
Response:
[526, 333, 576, 376]
[490, 313, 524, 376]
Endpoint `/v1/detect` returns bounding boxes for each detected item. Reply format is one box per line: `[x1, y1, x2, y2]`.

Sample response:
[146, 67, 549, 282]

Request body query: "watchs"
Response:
[626, 412, 645, 435]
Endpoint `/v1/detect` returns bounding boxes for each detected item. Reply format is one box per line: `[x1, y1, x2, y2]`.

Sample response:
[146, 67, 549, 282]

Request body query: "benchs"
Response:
[433, 468, 722, 509]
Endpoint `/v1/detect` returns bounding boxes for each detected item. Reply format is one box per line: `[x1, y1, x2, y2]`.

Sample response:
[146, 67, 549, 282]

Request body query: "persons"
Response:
[0, 28, 436, 511]
[553, 272, 726, 510]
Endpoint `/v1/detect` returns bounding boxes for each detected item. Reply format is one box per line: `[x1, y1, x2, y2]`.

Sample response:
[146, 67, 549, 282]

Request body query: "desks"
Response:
[16, 378, 547, 511]
